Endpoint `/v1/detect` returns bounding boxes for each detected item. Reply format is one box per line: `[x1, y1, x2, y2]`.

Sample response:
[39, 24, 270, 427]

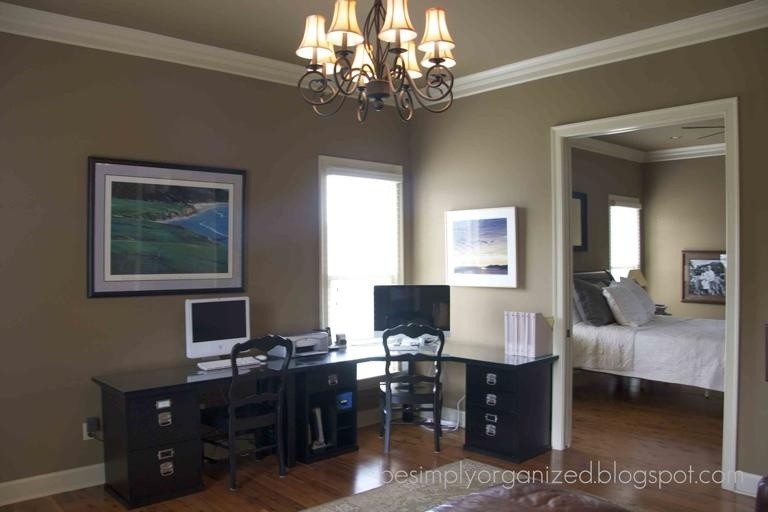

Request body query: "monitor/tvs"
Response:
[183, 294, 252, 361]
[373, 283, 451, 344]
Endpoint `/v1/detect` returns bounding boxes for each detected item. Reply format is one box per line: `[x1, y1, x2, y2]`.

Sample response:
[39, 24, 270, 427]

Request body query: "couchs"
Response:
[424, 478, 632, 512]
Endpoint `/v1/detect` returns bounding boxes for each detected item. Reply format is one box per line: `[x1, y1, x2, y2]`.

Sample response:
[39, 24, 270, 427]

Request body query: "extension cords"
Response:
[424, 417, 456, 429]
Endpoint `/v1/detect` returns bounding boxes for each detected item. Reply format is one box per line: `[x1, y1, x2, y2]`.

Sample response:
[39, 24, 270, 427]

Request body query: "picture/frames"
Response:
[680, 251, 726, 305]
[86, 157, 248, 299]
[571, 192, 587, 252]
[445, 206, 519, 290]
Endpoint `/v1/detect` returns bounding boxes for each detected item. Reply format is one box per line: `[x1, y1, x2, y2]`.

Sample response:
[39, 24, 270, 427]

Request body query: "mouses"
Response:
[257, 354, 267, 362]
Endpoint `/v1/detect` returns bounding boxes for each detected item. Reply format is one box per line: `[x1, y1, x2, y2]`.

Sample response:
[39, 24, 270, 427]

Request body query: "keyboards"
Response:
[387, 343, 419, 353]
[196, 357, 259, 370]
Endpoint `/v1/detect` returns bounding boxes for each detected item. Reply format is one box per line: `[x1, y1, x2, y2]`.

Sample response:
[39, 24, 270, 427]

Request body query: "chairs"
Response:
[201, 335, 293, 491]
[379, 323, 444, 454]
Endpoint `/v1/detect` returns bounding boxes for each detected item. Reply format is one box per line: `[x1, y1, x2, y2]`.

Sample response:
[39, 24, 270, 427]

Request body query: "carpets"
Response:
[298, 459, 647, 512]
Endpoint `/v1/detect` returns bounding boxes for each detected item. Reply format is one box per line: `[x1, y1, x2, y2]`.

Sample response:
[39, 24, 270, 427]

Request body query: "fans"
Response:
[682, 124, 725, 139]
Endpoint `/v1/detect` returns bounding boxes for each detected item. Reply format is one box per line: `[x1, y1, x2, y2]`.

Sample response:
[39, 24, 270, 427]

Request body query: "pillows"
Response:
[572, 276, 656, 327]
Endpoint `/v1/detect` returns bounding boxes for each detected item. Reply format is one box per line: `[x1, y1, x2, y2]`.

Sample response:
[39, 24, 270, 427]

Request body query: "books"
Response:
[504, 311, 552, 358]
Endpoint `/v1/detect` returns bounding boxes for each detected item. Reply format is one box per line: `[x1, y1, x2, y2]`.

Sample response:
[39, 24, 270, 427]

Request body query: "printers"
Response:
[266, 329, 330, 358]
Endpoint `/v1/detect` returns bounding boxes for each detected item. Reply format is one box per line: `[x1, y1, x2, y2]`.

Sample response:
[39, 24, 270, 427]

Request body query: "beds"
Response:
[570, 269, 726, 399]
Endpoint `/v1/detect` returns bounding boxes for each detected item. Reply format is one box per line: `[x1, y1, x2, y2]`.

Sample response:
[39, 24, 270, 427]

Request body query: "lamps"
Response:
[295, 0, 457, 125]
[628, 269, 647, 288]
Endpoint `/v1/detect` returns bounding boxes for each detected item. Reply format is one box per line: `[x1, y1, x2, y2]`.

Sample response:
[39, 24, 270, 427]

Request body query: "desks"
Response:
[92, 341, 559, 510]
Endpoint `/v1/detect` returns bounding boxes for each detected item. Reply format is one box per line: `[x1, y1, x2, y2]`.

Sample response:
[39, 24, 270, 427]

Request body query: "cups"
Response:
[336, 334, 345, 346]
